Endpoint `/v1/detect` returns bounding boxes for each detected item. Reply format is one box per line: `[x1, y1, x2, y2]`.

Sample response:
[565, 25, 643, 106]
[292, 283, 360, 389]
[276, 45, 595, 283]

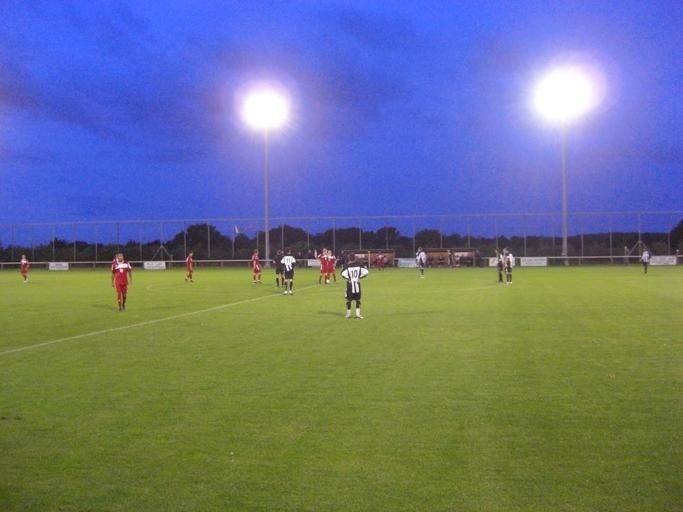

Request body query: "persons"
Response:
[273, 248, 384, 295]
[494, 247, 503, 282]
[640, 248, 650, 273]
[185, 251, 194, 283]
[20, 255, 29, 282]
[503, 247, 513, 284]
[340, 254, 369, 319]
[252, 249, 262, 283]
[111, 253, 132, 312]
[416, 246, 426, 278]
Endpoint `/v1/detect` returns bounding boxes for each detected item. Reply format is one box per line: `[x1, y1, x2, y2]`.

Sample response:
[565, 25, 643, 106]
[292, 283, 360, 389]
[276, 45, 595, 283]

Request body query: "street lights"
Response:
[238, 83, 289, 266]
[527, 70, 601, 256]
